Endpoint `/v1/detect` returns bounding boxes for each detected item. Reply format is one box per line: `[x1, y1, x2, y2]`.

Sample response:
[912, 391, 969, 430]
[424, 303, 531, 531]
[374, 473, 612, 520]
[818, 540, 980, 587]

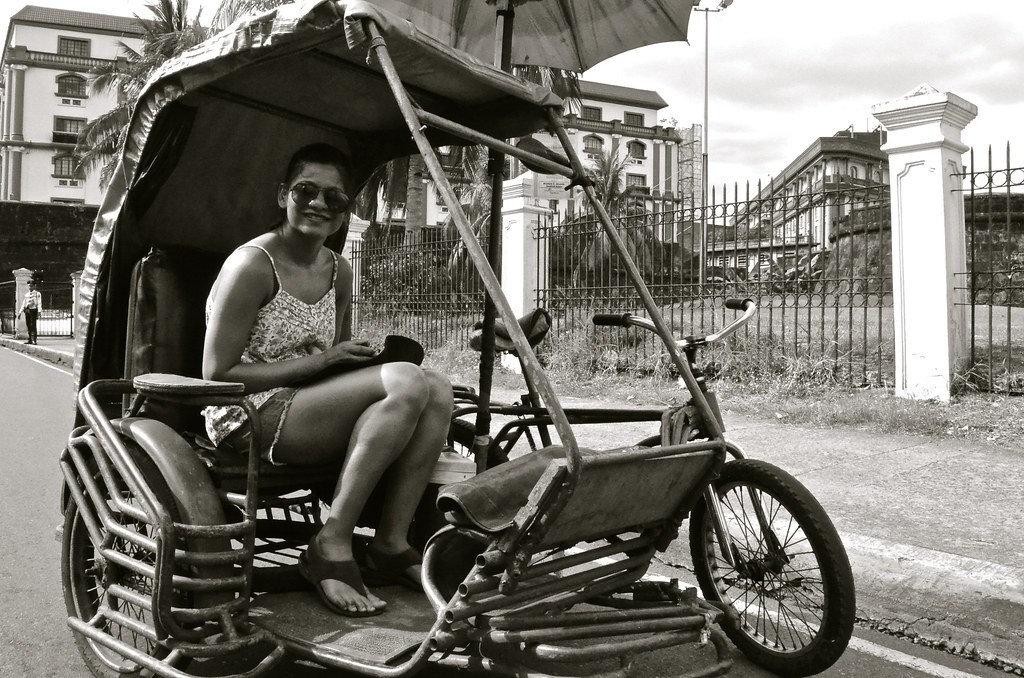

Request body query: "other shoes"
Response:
[33, 341, 38, 345]
[24, 341, 32, 344]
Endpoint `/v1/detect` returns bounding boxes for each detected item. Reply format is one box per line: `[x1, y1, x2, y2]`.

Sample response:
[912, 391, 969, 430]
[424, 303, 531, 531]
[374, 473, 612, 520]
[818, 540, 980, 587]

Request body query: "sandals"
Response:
[299, 533, 383, 616]
[365, 544, 433, 594]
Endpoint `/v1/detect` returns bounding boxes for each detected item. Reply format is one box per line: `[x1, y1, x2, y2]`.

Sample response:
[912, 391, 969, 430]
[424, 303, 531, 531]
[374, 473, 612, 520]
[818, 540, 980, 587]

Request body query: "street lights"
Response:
[692, 0, 733, 302]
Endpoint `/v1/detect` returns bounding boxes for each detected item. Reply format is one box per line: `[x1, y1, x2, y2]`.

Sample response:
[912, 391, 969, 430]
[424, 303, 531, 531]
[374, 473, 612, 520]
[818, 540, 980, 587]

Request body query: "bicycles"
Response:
[449, 295, 857, 678]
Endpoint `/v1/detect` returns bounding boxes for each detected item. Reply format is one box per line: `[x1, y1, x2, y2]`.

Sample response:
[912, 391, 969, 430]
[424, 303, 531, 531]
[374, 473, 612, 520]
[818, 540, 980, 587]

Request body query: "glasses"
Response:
[290, 180, 354, 213]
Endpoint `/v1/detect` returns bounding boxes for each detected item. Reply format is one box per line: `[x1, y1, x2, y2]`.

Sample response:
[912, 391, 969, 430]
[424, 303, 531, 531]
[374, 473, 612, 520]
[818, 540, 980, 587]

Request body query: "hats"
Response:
[27, 280, 36, 284]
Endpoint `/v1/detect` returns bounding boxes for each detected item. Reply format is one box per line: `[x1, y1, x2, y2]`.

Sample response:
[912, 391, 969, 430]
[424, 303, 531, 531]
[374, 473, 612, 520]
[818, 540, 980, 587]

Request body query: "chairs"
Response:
[121, 242, 340, 494]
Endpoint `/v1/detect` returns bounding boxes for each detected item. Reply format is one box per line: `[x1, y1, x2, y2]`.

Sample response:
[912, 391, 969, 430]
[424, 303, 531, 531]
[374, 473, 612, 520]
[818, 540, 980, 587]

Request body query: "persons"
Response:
[200, 142, 455, 618]
[17, 280, 42, 344]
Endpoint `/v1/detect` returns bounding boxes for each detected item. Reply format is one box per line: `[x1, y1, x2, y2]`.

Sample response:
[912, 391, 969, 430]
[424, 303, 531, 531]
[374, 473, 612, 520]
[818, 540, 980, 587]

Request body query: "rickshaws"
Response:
[55, 0, 727, 678]
